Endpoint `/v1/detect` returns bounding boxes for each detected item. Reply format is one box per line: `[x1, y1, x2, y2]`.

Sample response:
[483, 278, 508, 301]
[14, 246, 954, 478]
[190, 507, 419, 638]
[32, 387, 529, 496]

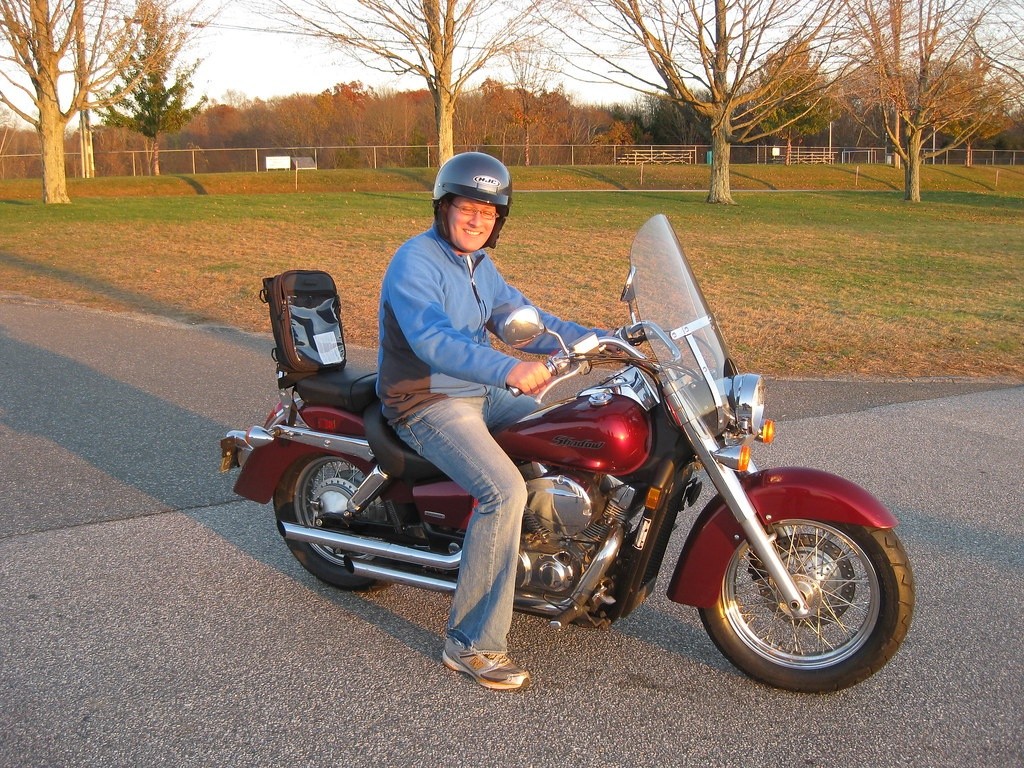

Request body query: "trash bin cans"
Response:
[706, 151, 713, 164]
[886, 153, 896, 164]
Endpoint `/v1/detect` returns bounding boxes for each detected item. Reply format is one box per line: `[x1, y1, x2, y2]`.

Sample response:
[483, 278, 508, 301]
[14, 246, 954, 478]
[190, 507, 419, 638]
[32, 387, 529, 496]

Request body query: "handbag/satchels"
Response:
[259, 269, 346, 426]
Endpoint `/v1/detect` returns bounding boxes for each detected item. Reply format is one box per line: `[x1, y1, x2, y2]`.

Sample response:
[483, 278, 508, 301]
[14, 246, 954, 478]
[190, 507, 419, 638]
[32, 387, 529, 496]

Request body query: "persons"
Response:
[375, 152, 628, 690]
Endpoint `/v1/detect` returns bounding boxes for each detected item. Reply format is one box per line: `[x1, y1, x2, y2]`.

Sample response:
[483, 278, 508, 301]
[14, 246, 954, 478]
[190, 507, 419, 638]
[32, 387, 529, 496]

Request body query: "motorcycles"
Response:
[218, 210, 918, 698]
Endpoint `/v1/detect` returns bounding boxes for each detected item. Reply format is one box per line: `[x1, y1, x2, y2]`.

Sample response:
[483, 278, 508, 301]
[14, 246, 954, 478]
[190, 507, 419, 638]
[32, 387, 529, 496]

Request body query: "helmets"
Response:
[432, 151, 513, 207]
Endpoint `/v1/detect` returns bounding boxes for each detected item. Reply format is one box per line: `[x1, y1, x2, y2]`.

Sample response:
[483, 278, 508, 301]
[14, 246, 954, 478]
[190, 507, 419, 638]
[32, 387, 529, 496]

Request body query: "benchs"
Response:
[769, 151, 838, 165]
[617, 150, 695, 165]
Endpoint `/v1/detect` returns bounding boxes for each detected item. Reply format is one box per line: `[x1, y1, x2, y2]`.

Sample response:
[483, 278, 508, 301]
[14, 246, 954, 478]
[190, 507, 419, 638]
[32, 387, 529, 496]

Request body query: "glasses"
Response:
[451, 201, 500, 220]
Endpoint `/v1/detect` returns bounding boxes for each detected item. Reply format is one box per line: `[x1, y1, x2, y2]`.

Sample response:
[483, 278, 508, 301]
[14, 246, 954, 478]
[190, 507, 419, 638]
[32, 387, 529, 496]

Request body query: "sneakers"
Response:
[443, 637, 530, 689]
